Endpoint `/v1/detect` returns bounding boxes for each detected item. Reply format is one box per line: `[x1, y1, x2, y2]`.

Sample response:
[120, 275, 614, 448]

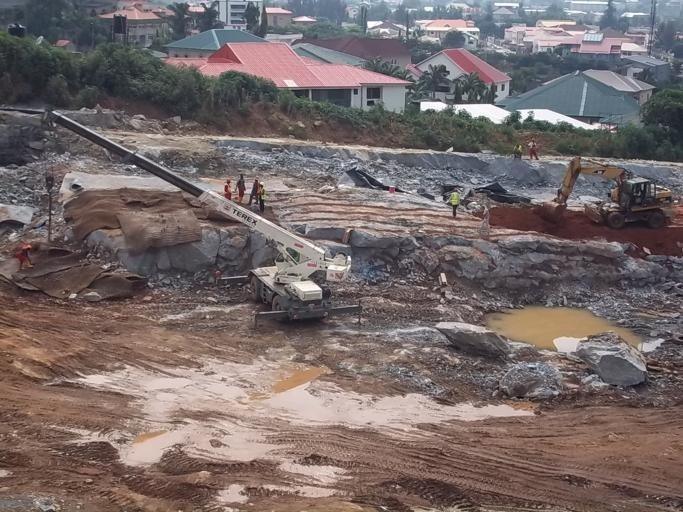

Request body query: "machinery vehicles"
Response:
[533, 156, 672, 229]
[40, 109, 362, 329]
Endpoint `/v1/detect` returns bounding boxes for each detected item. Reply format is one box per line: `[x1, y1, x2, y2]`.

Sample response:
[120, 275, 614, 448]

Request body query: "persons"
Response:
[512, 143, 522, 160]
[234, 173, 246, 203]
[14, 244, 35, 271]
[255, 184, 268, 213]
[444, 188, 460, 219]
[223, 178, 233, 200]
[527, 137, 539, 160]
[632, 186, 643, 203]
[245, 175, 259, 206]
